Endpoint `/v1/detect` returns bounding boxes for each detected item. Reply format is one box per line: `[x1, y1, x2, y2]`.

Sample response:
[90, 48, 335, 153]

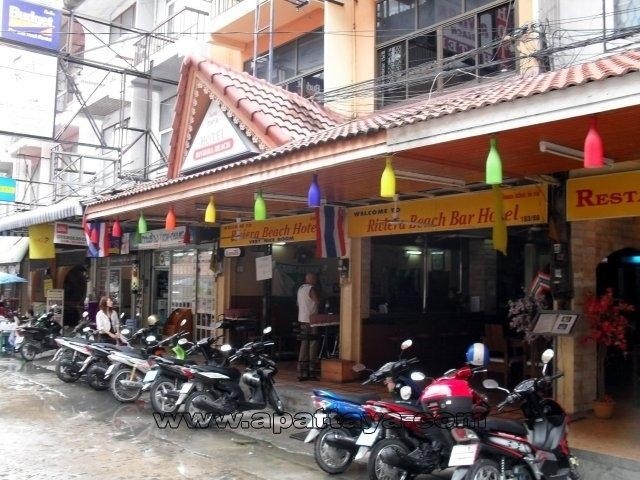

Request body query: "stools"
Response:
[387, 328, 474, 359]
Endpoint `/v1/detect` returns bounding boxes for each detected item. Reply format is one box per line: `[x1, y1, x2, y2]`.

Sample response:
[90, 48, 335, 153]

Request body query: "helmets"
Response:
[465, 342, 490, 367]
[147, 314, 161, 326]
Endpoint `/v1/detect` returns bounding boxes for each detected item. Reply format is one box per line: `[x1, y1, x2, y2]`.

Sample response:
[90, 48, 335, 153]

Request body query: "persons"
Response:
[94, 295, 121, 345]
[0, 302, 9, 318]
[293, 271, 322, 382]
[16, 309, 38, 329]
[109, 274, 119, 292]
[3, 308, 16, 323]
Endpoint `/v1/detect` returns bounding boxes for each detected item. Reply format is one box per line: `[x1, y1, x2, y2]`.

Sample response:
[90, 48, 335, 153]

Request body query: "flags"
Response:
[84, 222, 110, 258]
[314, 202, 348, 260]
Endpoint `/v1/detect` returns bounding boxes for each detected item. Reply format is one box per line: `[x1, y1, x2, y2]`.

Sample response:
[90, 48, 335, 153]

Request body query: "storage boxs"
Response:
[320, 357, 356, 383]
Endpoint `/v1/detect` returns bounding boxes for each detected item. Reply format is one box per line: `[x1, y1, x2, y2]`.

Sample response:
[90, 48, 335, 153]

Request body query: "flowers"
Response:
[579, 286, 636, 402]
[508, 283, 553, 381]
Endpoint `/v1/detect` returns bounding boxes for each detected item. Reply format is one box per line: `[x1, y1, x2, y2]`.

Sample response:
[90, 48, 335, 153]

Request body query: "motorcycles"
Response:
[0, 303, 290, 427]
[301, 335, 586, 480]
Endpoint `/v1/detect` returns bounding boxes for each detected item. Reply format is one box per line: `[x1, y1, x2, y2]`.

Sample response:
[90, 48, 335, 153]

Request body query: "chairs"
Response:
[216, 306, 340, 376]
[483, 323, 529, 390]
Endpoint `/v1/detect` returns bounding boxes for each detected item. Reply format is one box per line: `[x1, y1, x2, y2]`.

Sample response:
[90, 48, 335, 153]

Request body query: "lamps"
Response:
[88, 114, 619, 246]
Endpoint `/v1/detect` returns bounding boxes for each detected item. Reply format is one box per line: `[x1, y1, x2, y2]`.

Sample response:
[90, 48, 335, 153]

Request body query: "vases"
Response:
[591, 399, 616, 419]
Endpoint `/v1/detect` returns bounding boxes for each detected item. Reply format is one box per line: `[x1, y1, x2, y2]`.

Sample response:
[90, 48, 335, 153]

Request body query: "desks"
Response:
[480, 336, 531, 379]
